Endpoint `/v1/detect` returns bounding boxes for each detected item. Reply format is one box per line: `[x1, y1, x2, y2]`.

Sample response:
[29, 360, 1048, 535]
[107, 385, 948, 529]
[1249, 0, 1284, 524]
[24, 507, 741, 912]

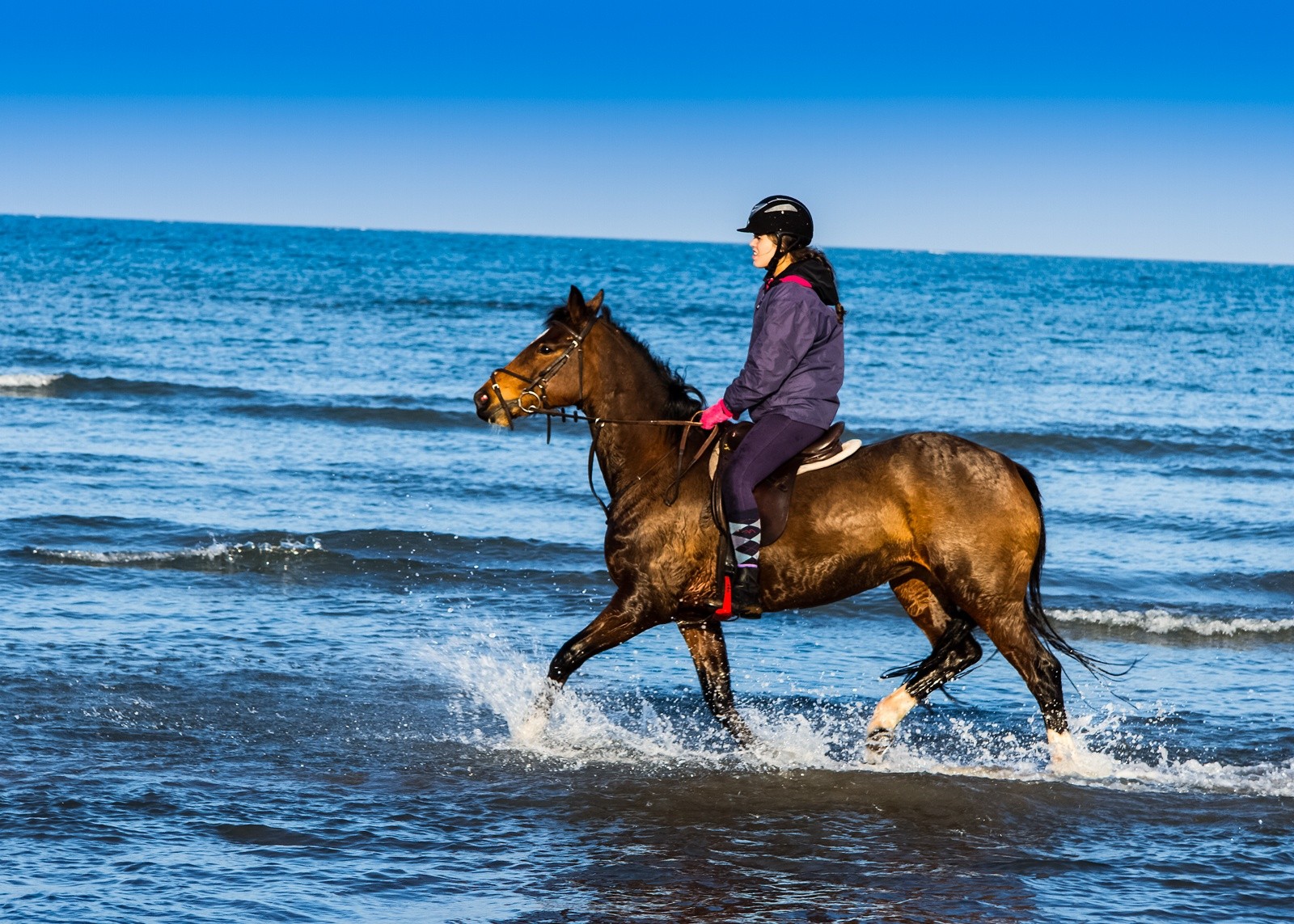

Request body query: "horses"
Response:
[474, 287, 1150, 776]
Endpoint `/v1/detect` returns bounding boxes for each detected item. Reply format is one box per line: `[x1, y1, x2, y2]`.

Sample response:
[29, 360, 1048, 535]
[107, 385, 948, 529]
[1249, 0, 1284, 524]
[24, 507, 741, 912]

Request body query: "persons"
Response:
[698, 196, 845, 619]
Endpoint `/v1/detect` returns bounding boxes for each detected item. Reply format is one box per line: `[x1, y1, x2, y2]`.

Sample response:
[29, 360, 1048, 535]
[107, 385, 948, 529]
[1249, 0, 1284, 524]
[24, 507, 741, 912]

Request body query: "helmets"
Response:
[736, 196, 824, 245]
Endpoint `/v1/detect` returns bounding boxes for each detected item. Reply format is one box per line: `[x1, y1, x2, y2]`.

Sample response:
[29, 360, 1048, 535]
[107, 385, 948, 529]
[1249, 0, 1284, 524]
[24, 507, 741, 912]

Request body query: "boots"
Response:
[704, 566, 763, 620]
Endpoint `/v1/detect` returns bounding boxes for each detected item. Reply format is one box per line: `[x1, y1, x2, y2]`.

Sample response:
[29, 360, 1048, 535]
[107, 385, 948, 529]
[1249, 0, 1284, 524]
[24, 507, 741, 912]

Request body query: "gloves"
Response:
[699, 399, 747, 434]
